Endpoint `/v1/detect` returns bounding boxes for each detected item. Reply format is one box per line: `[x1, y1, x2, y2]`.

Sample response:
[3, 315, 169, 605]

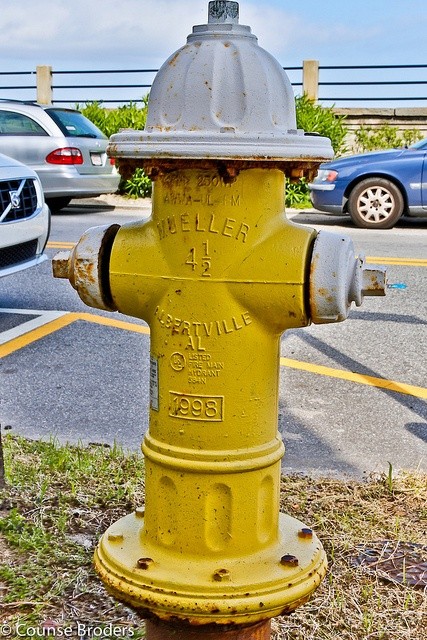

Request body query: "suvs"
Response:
[0, 102, 121, 212]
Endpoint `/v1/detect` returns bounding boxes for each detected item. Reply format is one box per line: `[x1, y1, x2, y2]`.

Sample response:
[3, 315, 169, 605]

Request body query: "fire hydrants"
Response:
[51, 0, 386, 640]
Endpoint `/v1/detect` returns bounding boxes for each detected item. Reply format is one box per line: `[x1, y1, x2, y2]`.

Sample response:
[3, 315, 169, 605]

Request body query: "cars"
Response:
[0, 153, 51, 278]
[308, 136, 427, 229]
